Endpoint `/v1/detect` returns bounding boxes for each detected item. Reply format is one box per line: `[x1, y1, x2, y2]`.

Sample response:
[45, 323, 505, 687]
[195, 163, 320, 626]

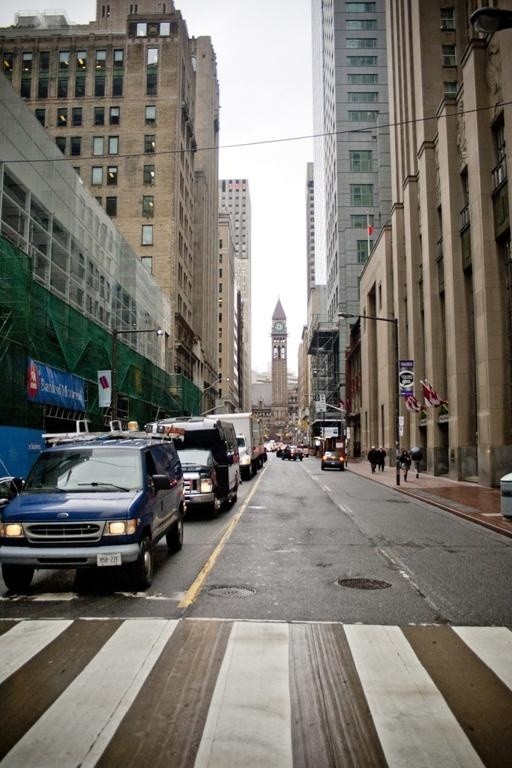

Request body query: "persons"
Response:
[368, 446, 378, 474]
[409, 450, 423, 478]
[377, 446, 386, 472]
[400, 450, 411, 482]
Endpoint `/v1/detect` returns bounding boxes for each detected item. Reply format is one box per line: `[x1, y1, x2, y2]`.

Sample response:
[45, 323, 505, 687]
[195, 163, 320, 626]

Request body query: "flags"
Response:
[405, 383, 448, 413]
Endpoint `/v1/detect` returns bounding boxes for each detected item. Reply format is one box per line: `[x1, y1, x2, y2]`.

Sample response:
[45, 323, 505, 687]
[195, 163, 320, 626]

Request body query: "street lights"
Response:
[336, 310, 405, 488]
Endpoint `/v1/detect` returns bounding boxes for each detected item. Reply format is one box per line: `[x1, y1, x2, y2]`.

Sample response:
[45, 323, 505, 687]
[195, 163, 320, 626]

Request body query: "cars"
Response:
[321, 451, 345, 470]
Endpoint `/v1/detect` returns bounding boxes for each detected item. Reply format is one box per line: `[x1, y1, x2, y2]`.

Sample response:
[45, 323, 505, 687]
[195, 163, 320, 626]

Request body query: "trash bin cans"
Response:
[501, 472, 511, 523]
[353, 441, 362, 457]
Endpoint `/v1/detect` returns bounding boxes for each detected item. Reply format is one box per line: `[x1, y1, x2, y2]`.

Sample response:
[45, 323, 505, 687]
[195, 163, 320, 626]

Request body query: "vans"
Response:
[141, 415, 241, 516]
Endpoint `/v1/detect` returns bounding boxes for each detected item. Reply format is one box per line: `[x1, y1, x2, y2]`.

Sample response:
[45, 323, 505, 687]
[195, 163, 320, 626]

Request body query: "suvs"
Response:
[0, 437, 187, 593]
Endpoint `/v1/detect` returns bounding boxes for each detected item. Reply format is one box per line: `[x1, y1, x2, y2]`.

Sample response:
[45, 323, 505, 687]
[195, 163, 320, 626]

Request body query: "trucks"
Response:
[207, 411, 266, 482]
